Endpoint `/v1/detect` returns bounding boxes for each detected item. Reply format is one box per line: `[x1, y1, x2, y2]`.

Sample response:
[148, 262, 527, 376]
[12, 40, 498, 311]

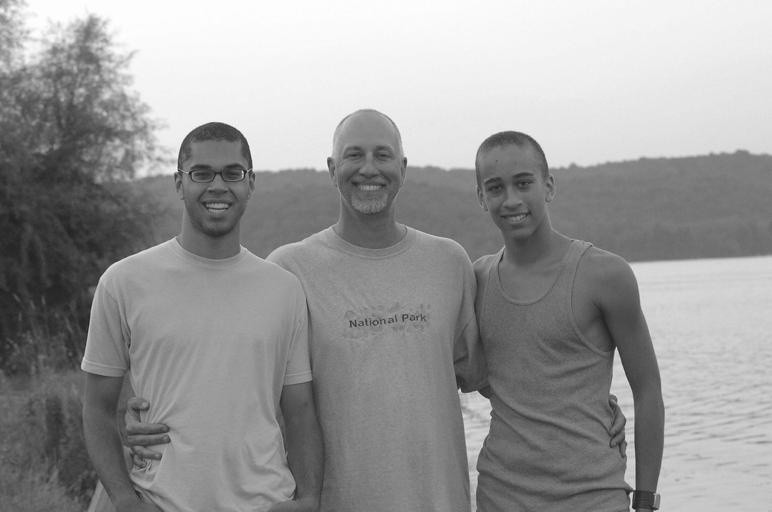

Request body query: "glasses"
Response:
[177, 166, 252, 183]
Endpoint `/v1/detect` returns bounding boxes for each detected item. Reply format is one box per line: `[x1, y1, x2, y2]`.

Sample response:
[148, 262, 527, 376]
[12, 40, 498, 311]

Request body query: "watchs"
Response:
[631, 490, 661, 510]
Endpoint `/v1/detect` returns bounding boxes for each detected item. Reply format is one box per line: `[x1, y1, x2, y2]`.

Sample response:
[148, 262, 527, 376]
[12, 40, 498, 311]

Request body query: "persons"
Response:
[455, 131, 665, 512]
[81, 122, 325, 512]
[124, 108, 628, 511]
